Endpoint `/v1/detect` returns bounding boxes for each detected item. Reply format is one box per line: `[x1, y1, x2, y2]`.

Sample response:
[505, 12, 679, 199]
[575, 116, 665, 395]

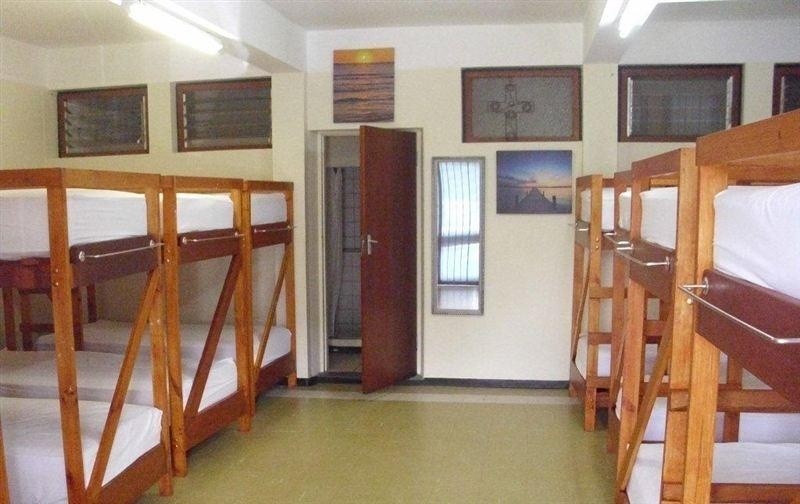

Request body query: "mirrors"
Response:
[430, 156, 486, 316]
